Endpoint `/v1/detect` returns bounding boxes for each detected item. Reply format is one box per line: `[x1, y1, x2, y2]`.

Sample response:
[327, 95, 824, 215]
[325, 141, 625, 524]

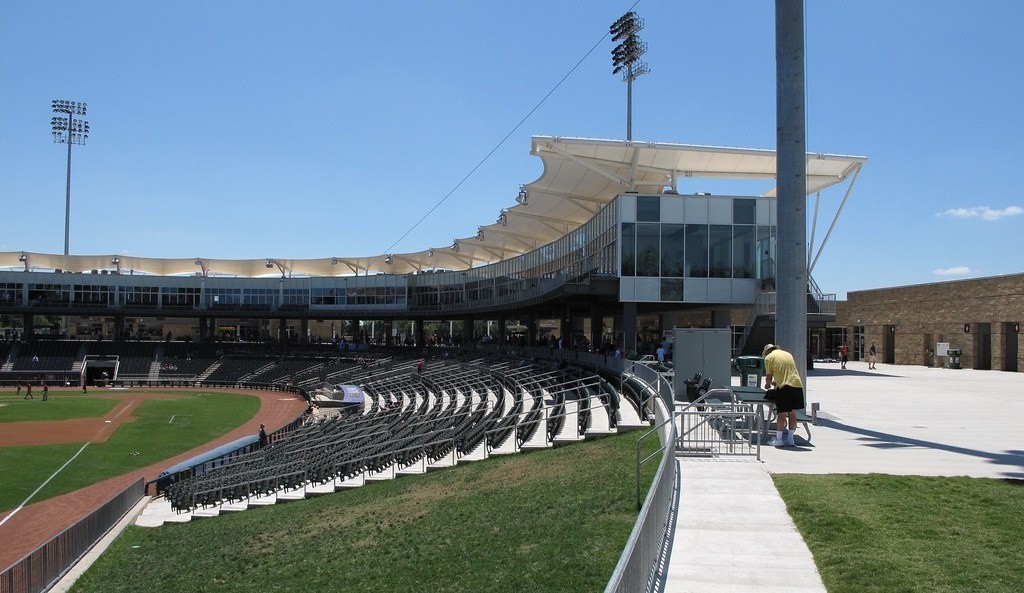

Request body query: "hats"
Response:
[762, 344, 780, 358]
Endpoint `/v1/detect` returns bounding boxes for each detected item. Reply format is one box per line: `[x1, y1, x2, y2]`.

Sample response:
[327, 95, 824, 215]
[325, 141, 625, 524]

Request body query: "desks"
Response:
[722, 385, 777, 443]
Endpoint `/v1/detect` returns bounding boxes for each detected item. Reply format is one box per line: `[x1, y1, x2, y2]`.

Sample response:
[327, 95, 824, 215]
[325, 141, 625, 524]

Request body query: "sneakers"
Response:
[785, 439, 794, 445]
[767, 436, 784, 446]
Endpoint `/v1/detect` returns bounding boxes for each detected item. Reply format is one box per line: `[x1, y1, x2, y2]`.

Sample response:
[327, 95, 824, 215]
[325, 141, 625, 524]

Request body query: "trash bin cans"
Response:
[736, 355, 767, 388]
[947, 349, 962, 369]
[838, 346, 848, 361]
[105, 379, 110, 385]
[629, 351, 638, 360]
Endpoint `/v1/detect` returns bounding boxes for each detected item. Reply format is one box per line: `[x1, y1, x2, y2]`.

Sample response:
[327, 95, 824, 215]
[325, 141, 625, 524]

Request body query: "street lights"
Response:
[610, 12, 651, 142]
[50, 100, 90, 255]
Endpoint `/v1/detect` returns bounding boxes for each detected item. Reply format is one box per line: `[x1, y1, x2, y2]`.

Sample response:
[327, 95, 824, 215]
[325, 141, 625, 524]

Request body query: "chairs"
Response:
[684, 373, 714, 409]
[1, 341, 620, 514]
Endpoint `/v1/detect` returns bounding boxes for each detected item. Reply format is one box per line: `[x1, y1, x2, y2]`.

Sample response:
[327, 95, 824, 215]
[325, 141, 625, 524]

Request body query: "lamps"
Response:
[195, 260, 202, 265]
[1015, 323, 1019, 332]
[519, 190, 527, 205]
[498, 214, 506, 225]
[452, 243, 459, 251]
[429, 251, 433, 257]
[385, 257, 390, 263]
[112, 257, 119, 263]
[331, 260, 337, 264]
[19, 254, 26, 261]
[477, 231, 484, 240]
[265, 260, 273, 267]
[965, 323, 970, 332]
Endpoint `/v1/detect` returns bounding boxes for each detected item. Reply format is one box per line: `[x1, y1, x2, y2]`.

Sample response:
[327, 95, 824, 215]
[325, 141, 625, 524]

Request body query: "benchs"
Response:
[756, 403, 812, 446]
[706, 399, 744, 433]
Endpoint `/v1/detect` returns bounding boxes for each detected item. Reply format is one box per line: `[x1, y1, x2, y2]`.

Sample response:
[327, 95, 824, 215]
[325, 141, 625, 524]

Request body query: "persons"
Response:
[423, 331, 623, 368]
[868, 339, 877, 370]
[83, 376, 87, 393]
[656, 336, 670, 362]
[0, 290, 51, 308]
[42, 383, 48, 401]
[0, 324, 173, 347]
[762, 343, 805, 446]
[24, 382, 33, 399]
[841, 342, 849, 369]
[33, 356, 38, 361]
[418, 360, 423, 373]
[204, 329, 411, 365]
[162, 363, 178, 372]
[259, 424, 268, 446]
[15, 377, 21, 394]
